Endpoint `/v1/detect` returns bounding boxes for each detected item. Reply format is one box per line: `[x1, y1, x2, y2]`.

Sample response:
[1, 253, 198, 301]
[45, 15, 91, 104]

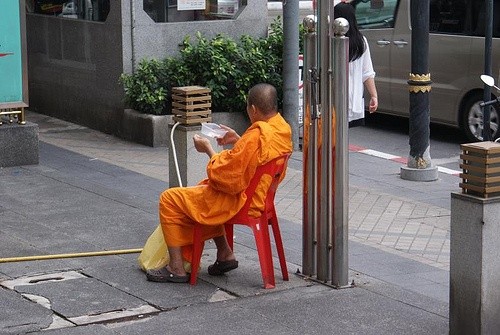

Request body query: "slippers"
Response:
[146, 265, 188, 283]
[207, 259, 238, 274]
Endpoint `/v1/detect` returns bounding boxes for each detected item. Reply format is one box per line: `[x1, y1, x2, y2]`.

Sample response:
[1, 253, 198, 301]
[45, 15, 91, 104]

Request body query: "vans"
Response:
[349, 0, 500, 140]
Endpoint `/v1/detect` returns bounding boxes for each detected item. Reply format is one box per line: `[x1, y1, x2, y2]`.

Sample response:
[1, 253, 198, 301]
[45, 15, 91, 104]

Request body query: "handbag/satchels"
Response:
[138, 223, 171, 272]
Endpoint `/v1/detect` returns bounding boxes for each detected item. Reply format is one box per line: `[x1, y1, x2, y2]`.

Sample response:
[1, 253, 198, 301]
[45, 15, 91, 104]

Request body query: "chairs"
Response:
[189, 153, 290, 288]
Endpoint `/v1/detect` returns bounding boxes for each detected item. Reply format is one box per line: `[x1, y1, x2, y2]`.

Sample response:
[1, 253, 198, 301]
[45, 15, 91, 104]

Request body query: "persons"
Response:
[333, 2, 378, 125]
[147, 85, 293, 282]
